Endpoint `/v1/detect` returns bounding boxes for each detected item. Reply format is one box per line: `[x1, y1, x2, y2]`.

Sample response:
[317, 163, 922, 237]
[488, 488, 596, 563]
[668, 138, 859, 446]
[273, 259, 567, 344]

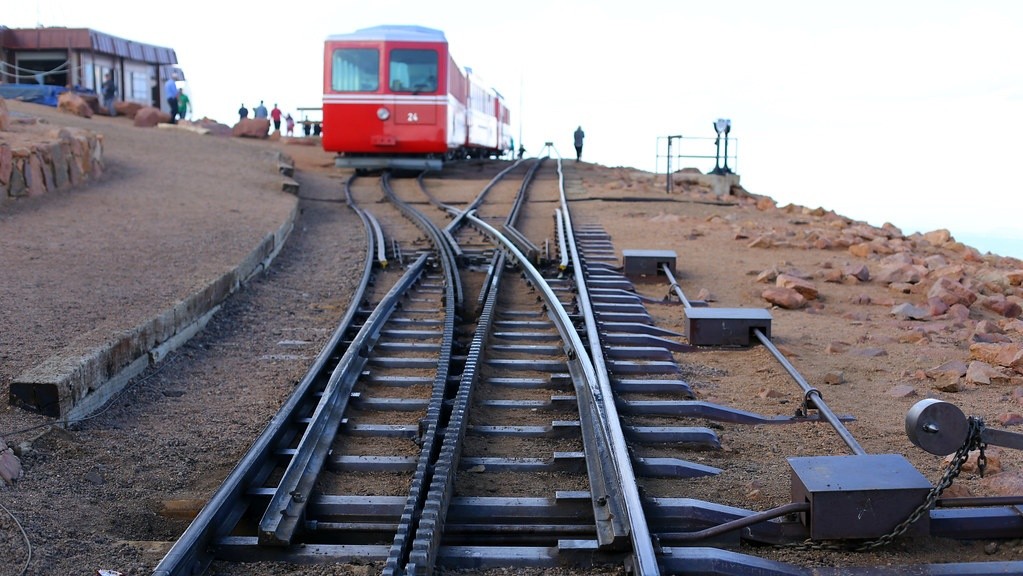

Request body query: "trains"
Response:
[321, 23, 513, 178]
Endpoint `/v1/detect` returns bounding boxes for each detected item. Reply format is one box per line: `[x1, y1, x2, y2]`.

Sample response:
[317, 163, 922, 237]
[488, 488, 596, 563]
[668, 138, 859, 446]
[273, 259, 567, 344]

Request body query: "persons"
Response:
[573, 126, 585, 162]
[271, 103, 284, 130]
[284, 113, 295, 137]
[164, 73, 179, 123]
[178, 88, 192, 119]
[253, 100, 268, 119]
[517, 144, 526, 159]
[313, 121, 321, 136]
[302, 115, 311, 136]
[101, 74, 116, 116]
[238, 104, 248, 121]
